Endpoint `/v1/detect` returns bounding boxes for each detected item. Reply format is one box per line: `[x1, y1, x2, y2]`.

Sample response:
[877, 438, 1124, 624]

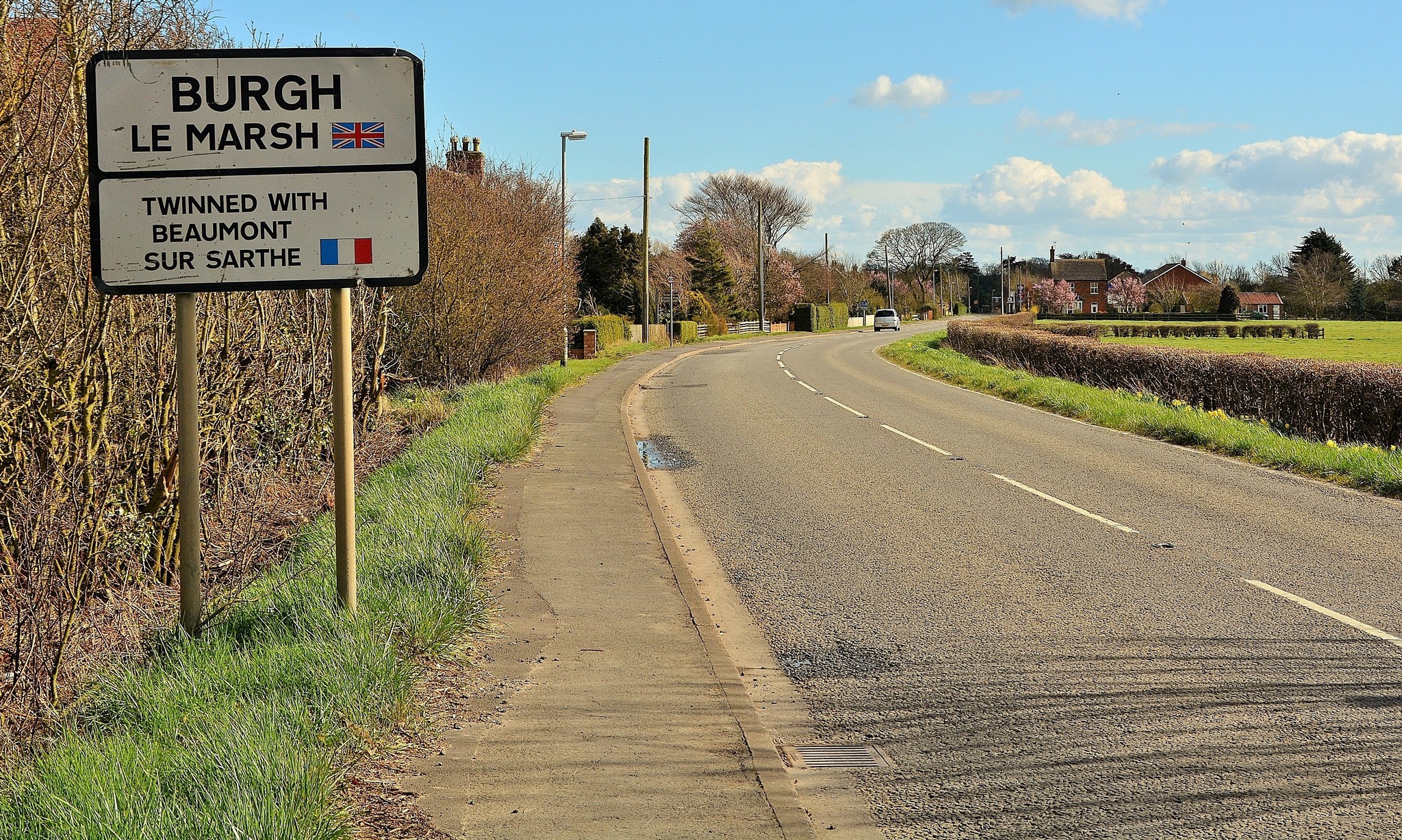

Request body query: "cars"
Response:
[1240, 309, 1270, 320]
[873, 308, 901, 331]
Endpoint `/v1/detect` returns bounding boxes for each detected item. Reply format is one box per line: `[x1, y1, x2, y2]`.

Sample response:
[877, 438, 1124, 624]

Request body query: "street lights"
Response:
[950, 282, 955, 315]
[968, 287, 973, 314]
[559, 130, 588, 370]
[992, 289, 995, 297]
[933, 269, 938, 305]
